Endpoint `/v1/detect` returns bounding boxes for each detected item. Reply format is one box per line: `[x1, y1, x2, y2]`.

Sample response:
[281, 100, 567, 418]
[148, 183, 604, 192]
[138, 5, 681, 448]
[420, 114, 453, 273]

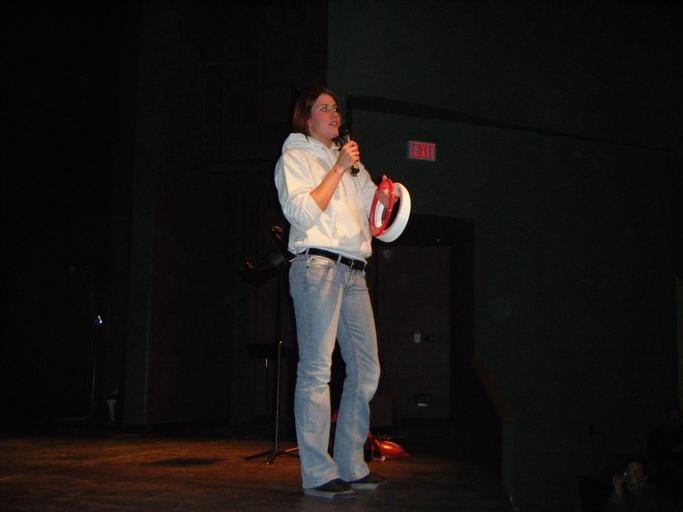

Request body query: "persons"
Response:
[271, 83, 402, 500]
[603, 455, 657, 511]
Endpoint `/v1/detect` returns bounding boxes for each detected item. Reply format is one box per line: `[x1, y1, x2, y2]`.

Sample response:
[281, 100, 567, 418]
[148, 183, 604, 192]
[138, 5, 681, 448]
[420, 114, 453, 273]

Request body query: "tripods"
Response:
[245, 270, 299, 466]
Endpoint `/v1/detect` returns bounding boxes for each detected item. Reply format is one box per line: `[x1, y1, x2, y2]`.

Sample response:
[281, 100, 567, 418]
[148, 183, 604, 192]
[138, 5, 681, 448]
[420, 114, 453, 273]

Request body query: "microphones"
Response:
[338, 125, 360, 175]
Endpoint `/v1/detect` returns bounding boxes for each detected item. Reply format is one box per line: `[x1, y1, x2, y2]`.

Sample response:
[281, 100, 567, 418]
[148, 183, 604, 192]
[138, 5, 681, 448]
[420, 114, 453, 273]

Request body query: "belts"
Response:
[301, 248, 368, 271]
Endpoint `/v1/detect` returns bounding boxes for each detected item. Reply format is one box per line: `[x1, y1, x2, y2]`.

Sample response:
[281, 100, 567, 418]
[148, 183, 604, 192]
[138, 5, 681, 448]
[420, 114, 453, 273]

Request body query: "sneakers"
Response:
[303, 478, 357, 500]
[347, 472, 388, 490]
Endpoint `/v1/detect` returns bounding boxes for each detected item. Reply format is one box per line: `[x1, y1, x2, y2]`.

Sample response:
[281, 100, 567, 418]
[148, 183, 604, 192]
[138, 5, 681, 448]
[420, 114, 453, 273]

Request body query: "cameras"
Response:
[622, 472, 629, 480]
